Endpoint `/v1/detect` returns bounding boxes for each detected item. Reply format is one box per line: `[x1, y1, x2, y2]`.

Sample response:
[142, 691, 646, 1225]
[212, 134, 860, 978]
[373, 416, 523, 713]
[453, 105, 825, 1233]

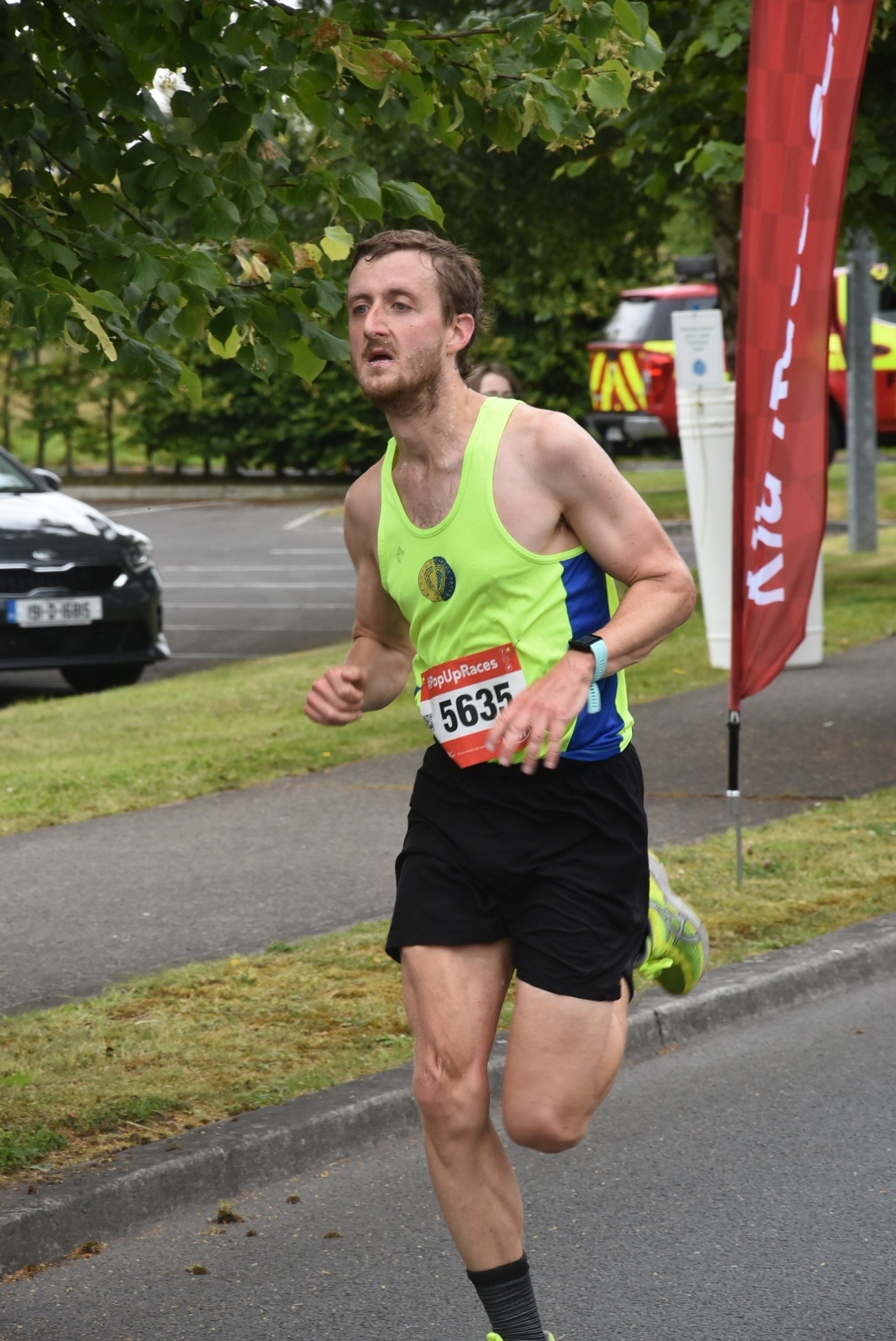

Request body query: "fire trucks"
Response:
[584, 253, 896, 466]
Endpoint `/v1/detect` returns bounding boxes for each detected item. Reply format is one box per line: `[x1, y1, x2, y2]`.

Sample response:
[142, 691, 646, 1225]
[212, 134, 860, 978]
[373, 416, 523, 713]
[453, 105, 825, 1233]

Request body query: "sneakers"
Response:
[631, 850, 707, 996]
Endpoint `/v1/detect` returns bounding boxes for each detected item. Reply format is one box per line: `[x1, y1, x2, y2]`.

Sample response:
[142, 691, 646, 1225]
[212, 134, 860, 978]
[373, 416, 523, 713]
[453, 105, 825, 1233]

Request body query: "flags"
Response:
[731, 1, 876, 699]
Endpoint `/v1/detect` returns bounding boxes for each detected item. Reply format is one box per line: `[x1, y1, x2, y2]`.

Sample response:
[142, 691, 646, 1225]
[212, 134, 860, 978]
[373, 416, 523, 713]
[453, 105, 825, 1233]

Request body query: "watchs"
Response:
[566, 632, 608, 715]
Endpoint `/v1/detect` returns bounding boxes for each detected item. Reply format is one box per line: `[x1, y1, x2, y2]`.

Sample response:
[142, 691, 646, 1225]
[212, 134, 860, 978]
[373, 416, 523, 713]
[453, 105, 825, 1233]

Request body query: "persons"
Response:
[301, 227, 710, 1340]
[464, 362, 518, 400]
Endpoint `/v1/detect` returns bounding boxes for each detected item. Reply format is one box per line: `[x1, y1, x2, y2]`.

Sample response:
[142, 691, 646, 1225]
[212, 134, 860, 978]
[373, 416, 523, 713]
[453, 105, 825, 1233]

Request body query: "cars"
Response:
[0, 447, 170, 692]
